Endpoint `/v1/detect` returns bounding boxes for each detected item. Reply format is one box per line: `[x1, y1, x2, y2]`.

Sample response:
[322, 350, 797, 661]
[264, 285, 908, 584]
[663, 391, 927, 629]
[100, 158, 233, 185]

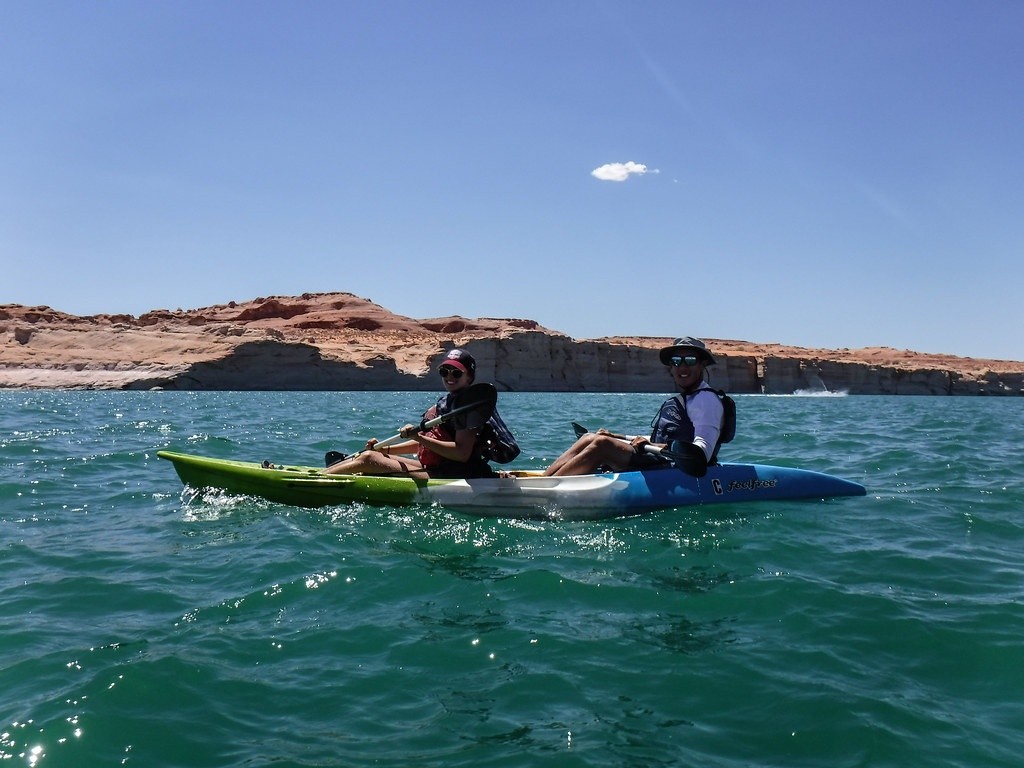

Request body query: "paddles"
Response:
[324, 383, 497, 468]
[571, 422, 707, 479]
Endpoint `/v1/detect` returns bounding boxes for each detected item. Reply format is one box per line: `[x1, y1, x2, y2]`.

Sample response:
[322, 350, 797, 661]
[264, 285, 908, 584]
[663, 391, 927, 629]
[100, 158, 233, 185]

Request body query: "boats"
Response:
[154, 449, 869, 518]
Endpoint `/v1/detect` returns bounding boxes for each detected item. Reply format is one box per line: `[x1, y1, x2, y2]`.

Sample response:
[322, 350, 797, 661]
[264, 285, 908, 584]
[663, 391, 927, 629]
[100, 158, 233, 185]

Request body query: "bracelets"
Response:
[387, 445, 390, 454]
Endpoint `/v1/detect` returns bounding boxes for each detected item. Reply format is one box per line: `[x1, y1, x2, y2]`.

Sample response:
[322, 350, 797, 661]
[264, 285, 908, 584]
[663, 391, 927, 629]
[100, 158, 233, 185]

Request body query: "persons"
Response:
[261, 348, 482, 479]
[500, 336, 725, 479]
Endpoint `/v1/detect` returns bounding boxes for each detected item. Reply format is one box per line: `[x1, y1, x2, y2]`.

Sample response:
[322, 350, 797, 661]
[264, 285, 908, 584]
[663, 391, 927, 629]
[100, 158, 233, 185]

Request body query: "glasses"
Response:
[439, 368, 463, 378]
[669, 355, 698, 367]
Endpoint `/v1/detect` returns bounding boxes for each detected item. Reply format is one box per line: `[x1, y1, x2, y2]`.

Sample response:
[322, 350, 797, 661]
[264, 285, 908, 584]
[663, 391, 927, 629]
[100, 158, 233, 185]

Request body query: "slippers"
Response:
[261, 460, 269, 468]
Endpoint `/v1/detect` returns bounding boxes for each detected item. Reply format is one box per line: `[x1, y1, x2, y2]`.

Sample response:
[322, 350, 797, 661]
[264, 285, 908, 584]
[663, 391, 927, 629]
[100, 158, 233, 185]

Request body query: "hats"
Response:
[436, 349, 475, 377]
[660, 336, 717, 366]
